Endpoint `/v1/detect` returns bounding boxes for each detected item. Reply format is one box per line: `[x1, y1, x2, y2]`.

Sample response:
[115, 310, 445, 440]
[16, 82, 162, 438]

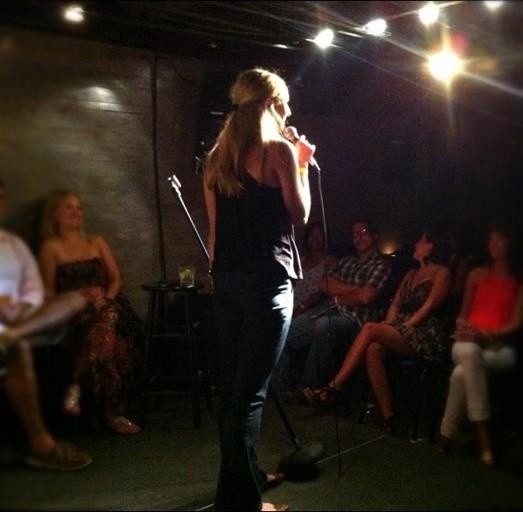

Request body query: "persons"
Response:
[0, 177, 95, 470]
[264, 213, 393, 407]
[433, 223, 522, 466]
[291, 220, 339, 319]
[37, 187, 141, 437]
[296, 228, 457, 436]
[200, 66, 317, 511]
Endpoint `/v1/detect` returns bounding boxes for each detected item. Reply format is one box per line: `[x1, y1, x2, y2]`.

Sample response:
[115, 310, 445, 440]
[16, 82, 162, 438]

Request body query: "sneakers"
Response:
[25, 440, 93, 471]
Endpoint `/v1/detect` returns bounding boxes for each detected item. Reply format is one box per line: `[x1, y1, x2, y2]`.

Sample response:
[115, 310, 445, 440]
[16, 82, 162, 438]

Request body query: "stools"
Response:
[137, 279, 215, 435]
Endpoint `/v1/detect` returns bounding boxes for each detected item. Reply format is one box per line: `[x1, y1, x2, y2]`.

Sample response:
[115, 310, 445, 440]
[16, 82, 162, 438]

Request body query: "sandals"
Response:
[302, 382, 345, 409]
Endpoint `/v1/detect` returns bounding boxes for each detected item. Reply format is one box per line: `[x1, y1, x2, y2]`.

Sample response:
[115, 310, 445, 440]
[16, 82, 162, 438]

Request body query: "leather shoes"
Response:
[260, 502, 289, 511]
[111, 419, 142, 435]
[266, 472, 285, 490]
[61, 392, 83, 416]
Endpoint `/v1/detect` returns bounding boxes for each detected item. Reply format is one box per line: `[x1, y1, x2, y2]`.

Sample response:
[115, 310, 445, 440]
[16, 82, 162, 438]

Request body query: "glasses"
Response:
[351, 226, 369, 239]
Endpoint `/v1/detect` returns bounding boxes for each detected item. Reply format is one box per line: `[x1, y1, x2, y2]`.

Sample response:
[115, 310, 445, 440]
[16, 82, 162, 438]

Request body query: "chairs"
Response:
[328, 332, 522, 455]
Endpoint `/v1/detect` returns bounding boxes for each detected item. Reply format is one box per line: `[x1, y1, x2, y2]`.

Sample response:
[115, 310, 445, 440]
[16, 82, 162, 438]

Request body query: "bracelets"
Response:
[207, 268, 213, 275]
[298, 167, 309, 176]
[298, 302, 306, 313]
[333, 295, 339, 306]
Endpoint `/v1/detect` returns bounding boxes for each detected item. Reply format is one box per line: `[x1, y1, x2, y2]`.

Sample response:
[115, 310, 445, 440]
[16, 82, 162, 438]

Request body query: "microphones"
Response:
[284, 125, 322, 171]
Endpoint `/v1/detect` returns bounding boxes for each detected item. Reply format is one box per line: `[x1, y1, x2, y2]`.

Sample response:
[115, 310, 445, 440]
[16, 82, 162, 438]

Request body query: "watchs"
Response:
[101, 296, 113, 306]
[487, 330, 495, 344]
